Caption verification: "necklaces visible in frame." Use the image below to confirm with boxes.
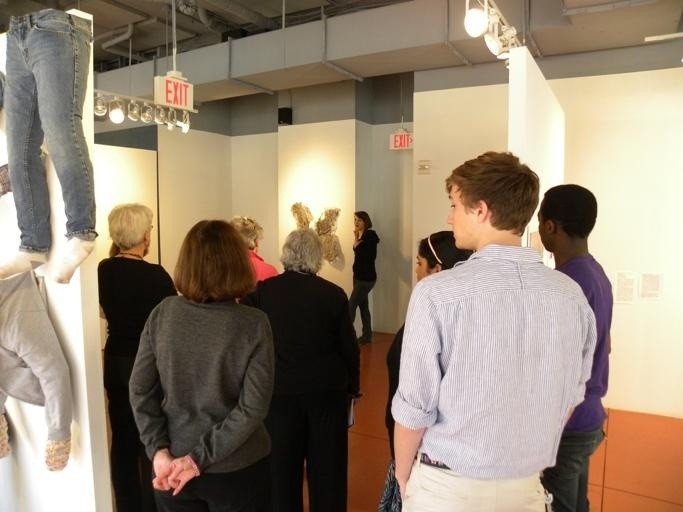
[119,252,143,259]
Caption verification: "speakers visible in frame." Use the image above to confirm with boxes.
[277,107,292,126]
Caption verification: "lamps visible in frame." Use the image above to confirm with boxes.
[464,0,518,70]
[93,90,191,134]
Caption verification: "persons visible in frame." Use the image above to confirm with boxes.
[129,219,275,510]
[97,204,178,512]
[378,230,474,511]
[227,216,279,306]
[243,228,360,510]
[391,151,598,512]
[106,240,149,510]
[347,211,379,344]
[538,184,614,512]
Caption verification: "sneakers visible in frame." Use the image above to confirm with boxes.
[357,337,371,344]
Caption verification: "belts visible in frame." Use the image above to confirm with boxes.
[413,452,449,470]
